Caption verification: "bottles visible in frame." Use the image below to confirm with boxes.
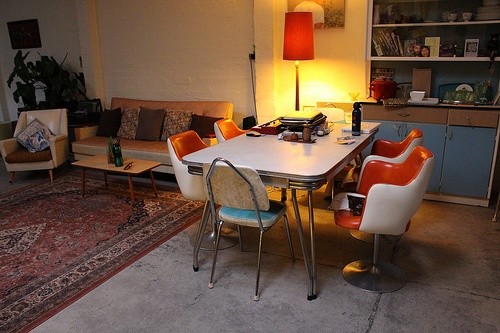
[351,102,362,136]
[302,124,310,142]
[112,141,123,167]
[291,132,298,140]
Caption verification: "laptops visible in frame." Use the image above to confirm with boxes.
[342,122,381,134]
[280,112,323,124]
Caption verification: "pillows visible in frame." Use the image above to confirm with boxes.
[97,106,224,141]
[16,118,56,153]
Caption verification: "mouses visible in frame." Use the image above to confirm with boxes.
[246,131,262,137]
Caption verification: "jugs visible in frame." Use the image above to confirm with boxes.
[475,80,490,102]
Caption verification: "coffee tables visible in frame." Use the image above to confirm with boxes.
[72,155,162,205]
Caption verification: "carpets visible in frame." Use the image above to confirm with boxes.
[0,170,222,333]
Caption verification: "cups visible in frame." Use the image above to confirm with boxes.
[409,90,426,102]
[462,13,472,22]
[446,13,457,22]
[443,90,475,101]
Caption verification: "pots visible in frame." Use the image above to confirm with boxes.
[369,77,399,100]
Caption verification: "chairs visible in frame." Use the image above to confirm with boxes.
[167,118,436,301]
[0,109,69,183]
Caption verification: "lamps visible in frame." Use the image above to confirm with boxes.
[283,12,314,111]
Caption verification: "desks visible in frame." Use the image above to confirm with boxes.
[182,123,380,301]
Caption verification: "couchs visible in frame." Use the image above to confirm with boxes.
[71,97,234,175]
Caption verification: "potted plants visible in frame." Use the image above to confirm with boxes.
[7,50,86,108]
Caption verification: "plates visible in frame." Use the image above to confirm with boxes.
[455,83,474,90]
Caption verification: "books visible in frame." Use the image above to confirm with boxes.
[373,28,404,57]
[278,110,328,128]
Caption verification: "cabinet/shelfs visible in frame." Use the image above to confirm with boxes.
[357,0,500,207]
